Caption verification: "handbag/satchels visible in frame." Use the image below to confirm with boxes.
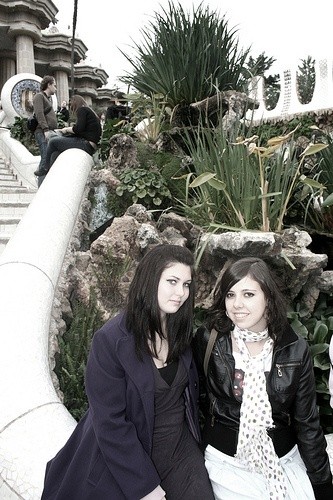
[27,113,39,132]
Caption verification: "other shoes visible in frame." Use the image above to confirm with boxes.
[34,168,47,176]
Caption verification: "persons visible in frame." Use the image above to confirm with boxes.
[57,107,69,122]
[41,244,213,500]
[33,75,58,188]
[34,95,102,176]
[104,92,131,120]
[193,257,333,500]
